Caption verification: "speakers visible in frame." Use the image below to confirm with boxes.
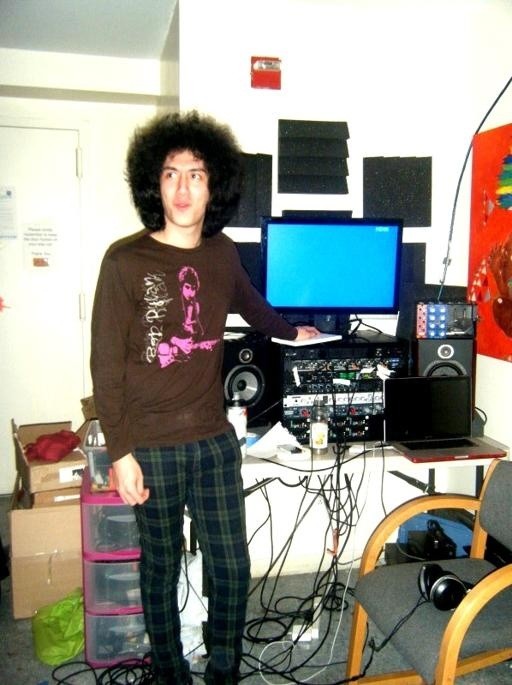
[222,338,280,428]
[413,339,477,423]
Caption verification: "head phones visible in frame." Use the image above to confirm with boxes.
[417,563,476,612]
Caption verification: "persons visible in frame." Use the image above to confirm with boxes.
[161,263,213,359]
[88,108,321,685]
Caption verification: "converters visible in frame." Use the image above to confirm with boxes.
[291,624,311,641]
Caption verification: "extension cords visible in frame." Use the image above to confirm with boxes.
[311,596,323,639]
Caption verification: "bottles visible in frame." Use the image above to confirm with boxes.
[224,392,248,460]
[308,399,329,456]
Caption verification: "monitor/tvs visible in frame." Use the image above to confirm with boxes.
[261,216,405,334]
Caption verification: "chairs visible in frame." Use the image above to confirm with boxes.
[350,456,510,683]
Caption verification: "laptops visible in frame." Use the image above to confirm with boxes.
[384,375,507,463]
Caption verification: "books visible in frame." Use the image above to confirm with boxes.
[272,332,343,349]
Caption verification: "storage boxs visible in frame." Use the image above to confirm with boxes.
[4,394,153,670]
